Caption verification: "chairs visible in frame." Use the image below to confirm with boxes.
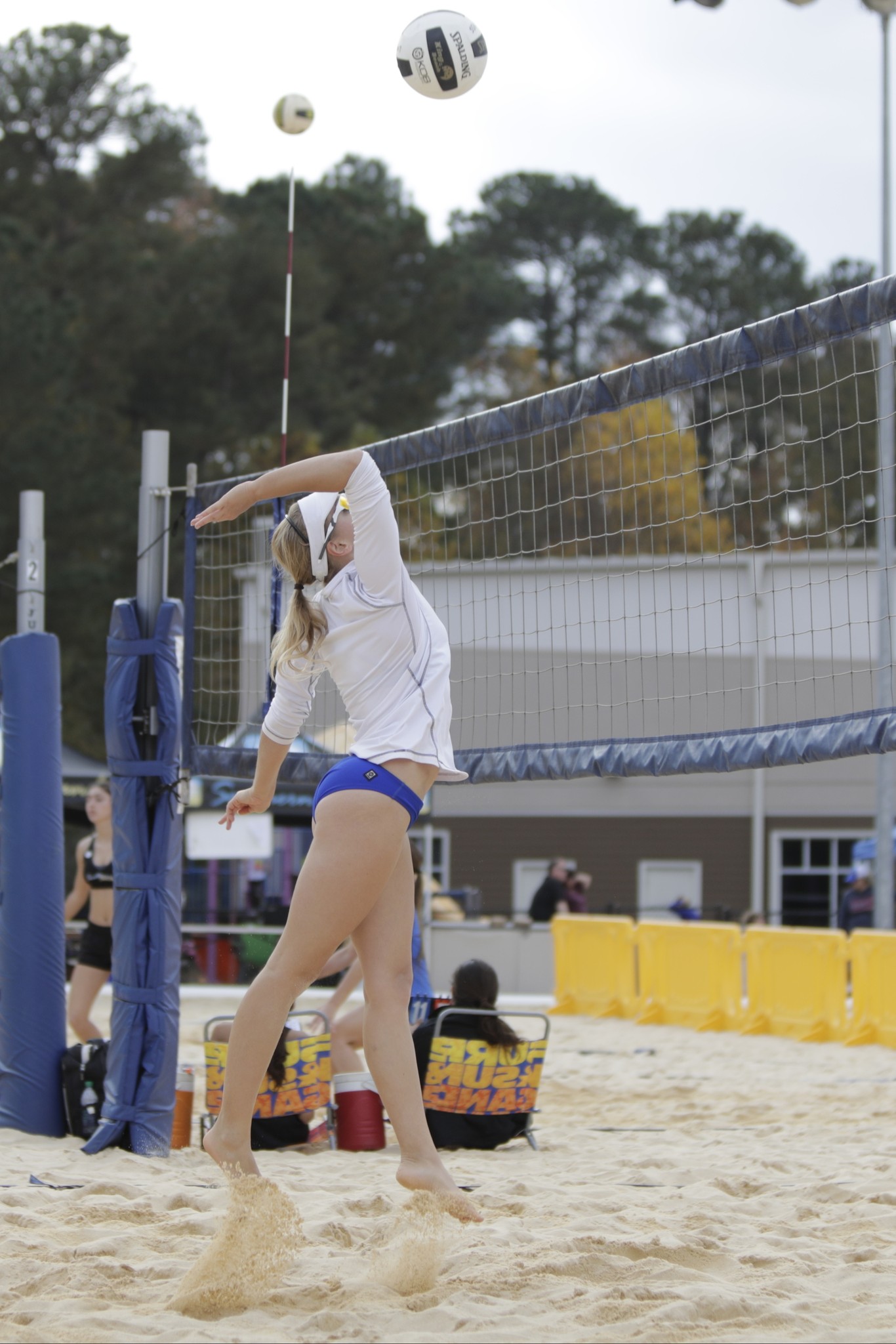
[423,1007,552,1153]
[199,1009,340,1154]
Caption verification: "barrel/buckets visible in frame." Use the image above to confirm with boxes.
[170,1067,194,1149]
[333,1072,386,1152]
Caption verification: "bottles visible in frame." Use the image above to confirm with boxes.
[81,1082,98,1132]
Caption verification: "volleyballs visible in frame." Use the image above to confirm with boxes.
[273,94,315,135]
[397,9,488,100]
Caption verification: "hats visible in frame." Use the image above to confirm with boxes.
[297,492,339,583]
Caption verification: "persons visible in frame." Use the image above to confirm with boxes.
[232,892,289,983]
[305,844,432,1075]
[836,861,873,931]
[411,958,529,1152]
[61,777,113,1044]
[527,855,593,923]
[667,895,699,919]
[189,450,485,1225]
[745,914,765,924]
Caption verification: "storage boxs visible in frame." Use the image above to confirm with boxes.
[333,1071,387,1152]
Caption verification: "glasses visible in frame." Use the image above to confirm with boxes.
[318,493,349,564]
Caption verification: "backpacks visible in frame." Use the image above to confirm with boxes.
[59,1038,111,1139]
